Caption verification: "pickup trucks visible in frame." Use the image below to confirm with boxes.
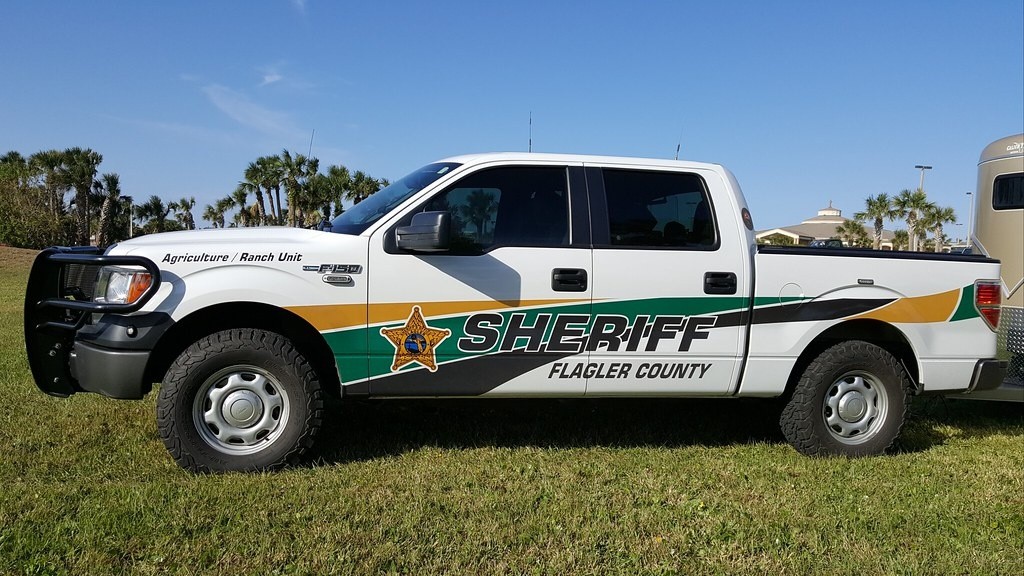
[22,152,1002,477]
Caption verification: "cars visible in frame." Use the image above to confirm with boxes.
[809,239,843,248]
[945,133,1024,402]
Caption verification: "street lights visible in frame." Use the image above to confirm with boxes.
[915,165,932,218]
[956,224,963,243]
[966,192,974,248]
